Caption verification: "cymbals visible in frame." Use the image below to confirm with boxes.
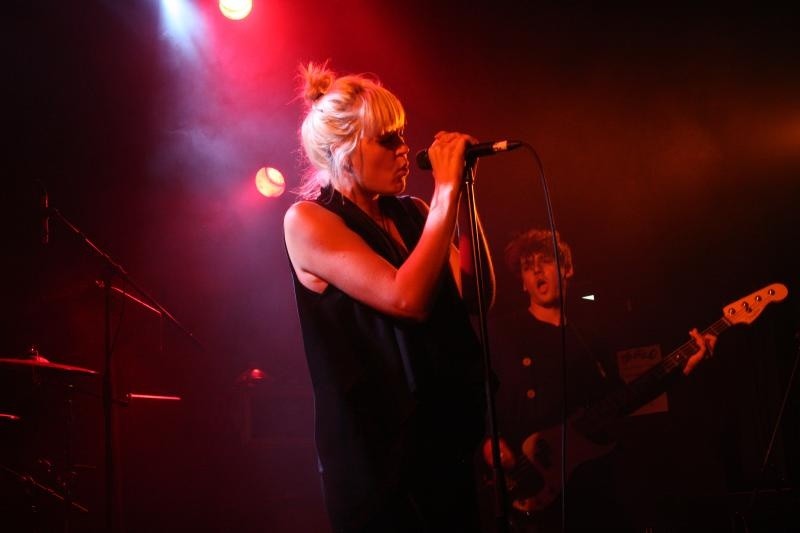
[0,355,100,375]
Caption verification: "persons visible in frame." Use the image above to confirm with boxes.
[282,60,497,532]
[486,229,707,533]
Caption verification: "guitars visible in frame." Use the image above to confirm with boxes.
[503,278,788,513]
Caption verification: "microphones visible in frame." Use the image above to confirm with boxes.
[417,140,522,170]
[40,187,49,243]
